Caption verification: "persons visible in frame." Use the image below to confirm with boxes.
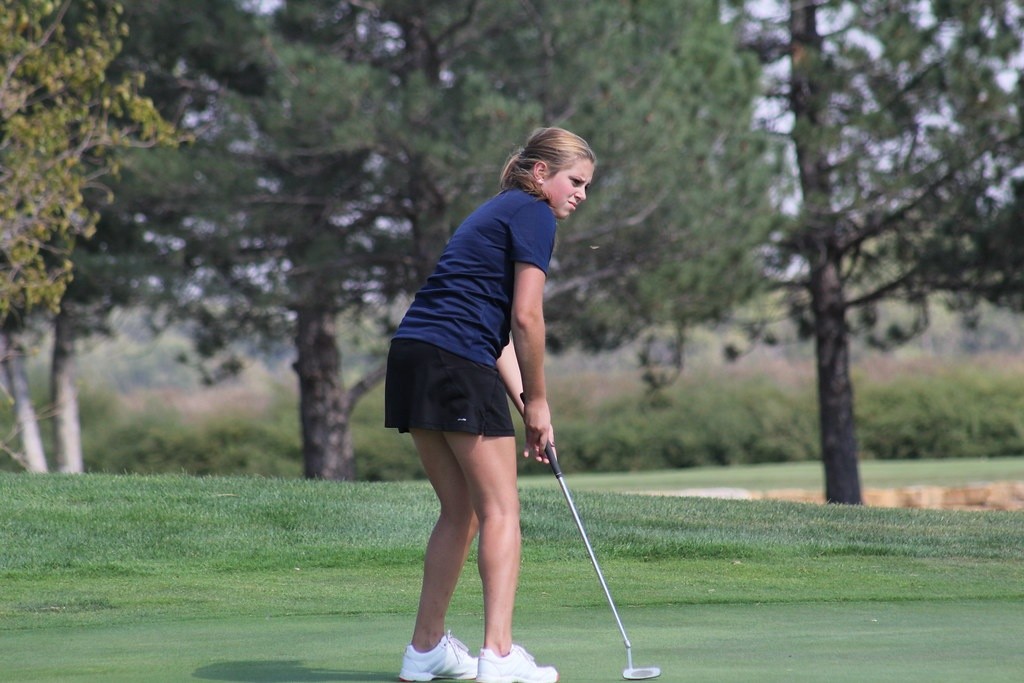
[384,127,596,683]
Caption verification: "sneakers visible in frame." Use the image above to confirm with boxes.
[397,628,480,682]
[476,644,559,683]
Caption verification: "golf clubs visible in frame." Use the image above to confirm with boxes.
[519,391,663,679]
